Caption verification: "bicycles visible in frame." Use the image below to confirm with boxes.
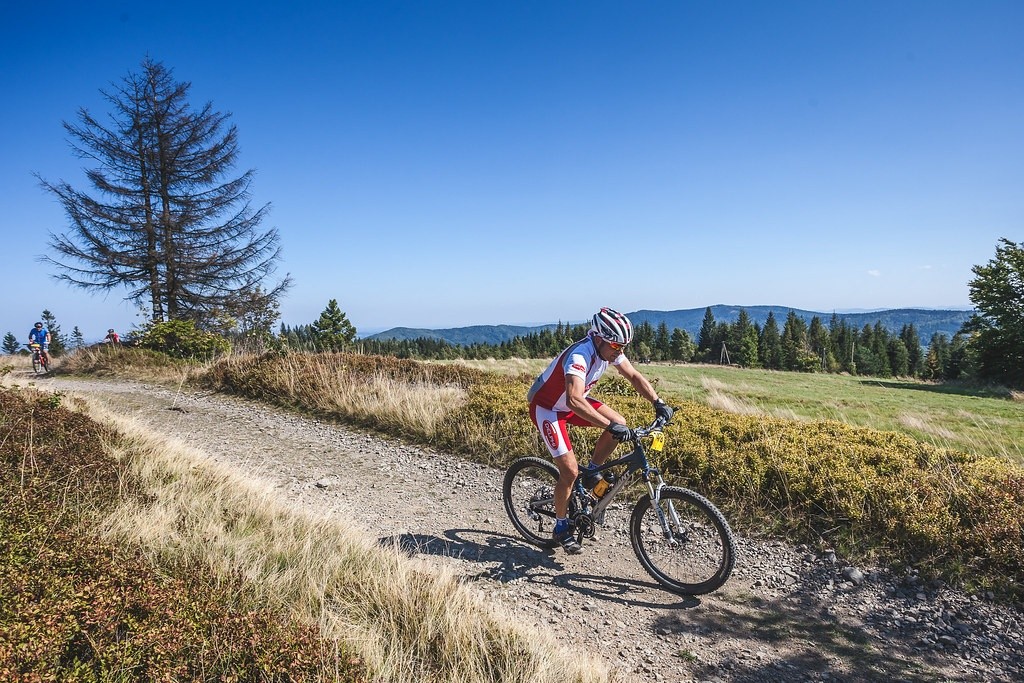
[503,407,736,597]
[29,341,50,374]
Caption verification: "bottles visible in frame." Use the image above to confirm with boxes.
[590,470,616,499]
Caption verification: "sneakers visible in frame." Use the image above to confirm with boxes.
[582,468,610,499]
[553,527,583,554]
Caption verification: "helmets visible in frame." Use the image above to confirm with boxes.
[588,307,634,345]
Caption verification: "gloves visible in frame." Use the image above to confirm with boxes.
[606,422,631,444]
[654,398,673,421]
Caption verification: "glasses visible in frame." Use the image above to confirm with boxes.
[610,343,623,351]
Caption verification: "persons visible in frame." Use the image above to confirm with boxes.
[102,328,119,343]
[524,305,675,556]
[28,322,51,365]
